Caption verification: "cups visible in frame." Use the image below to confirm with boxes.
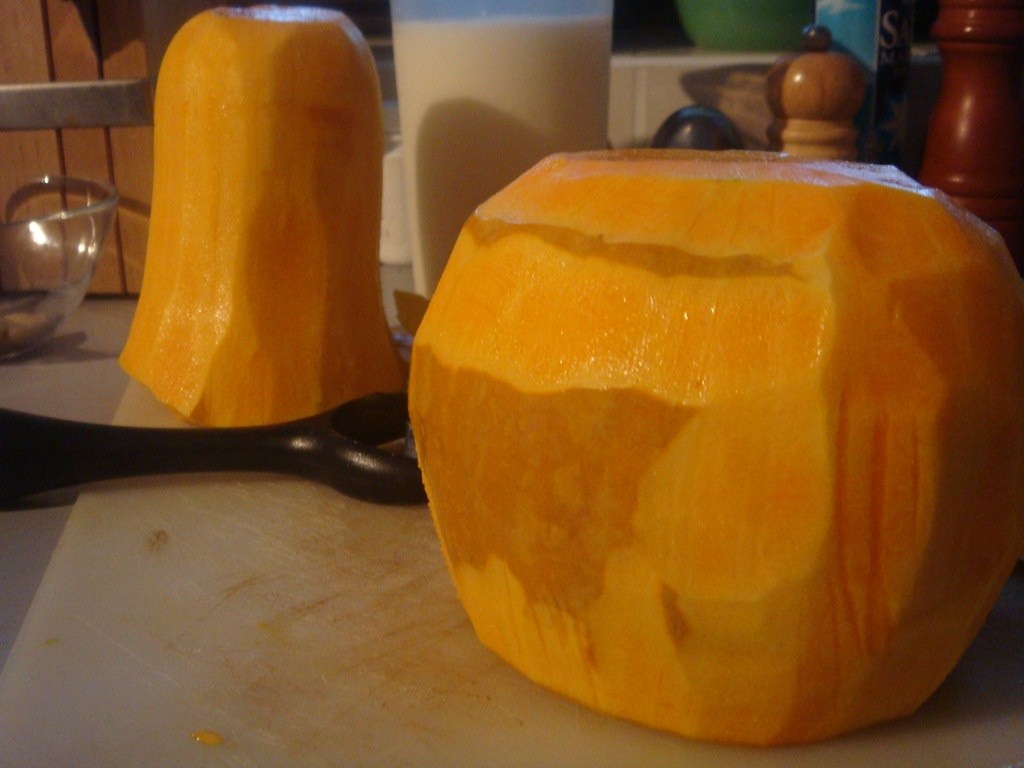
[387,0,612,297]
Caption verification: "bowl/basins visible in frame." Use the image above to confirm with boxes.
[675,0,814,50]
[0,167,121,362]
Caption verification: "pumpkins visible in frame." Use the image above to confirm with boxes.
[121,5,405,427]
[400,148,1024,745]
